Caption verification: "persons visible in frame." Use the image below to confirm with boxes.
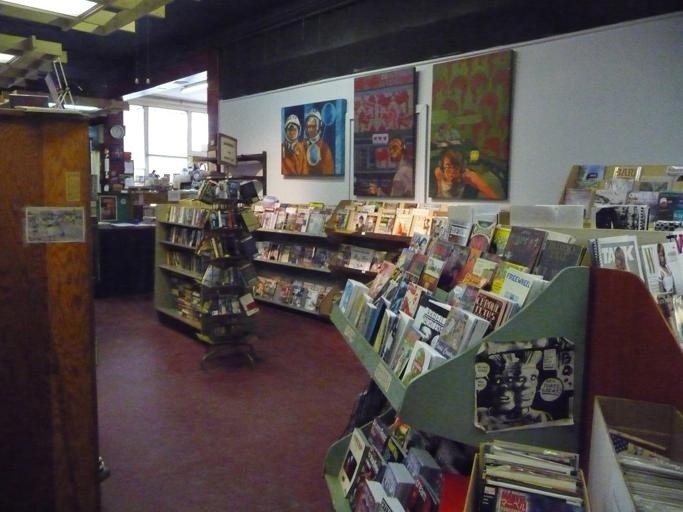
[432,152,495,201]
[353,214,365,236]
[654,244,681,328]
[512,352,554,423]
[300,107,337,174]
[611,244,629,270]
[366,136,412,195]
[280,114,308,177]
[297,212,307,232]
[476,353,515,428]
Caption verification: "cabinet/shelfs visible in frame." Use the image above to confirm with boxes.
[0,103,111,510]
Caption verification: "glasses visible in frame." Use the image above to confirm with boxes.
[441,165,460,174]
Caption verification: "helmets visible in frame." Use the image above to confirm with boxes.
[305,111,323,143]
[285,115,302,143]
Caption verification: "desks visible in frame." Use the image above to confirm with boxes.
[95,219,155,295]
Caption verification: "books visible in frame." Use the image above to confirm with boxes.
[335,213,586,512]
[249,198,449,316]
[563,163,683,340]
[161,165,261,360]
[610,426,681,512]
